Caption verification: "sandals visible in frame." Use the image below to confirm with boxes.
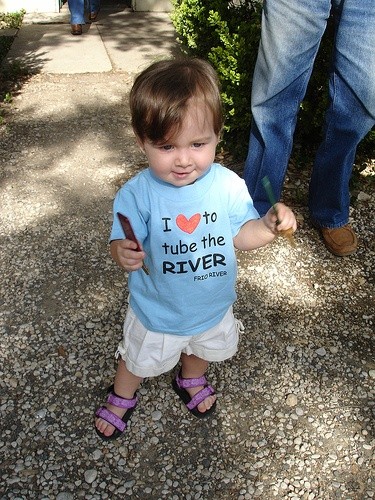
[172,368,218,418]
[95,385,138,439]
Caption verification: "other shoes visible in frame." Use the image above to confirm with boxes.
[320,226,357,256]
[88,8,100,19]
[71,24,82,35]
